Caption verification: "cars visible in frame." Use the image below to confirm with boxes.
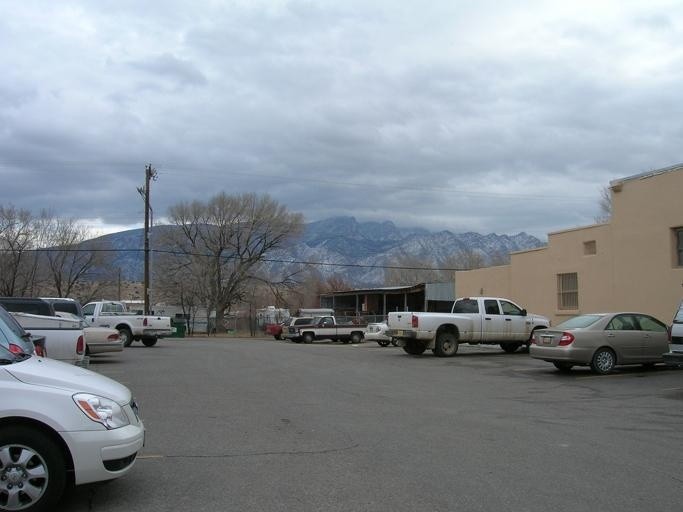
[0,296,124,371]
[0,344,147,511]
[529,312,670,376]
[663,298,682,368]
[364,317,406,347]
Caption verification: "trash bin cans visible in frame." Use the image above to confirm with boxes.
[171,318,186,338]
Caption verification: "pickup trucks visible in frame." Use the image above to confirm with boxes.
[262,308,367,344]
[80,299,177,347]
[385,297,551,357]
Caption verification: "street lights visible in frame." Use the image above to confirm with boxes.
[134,186,153,315]
